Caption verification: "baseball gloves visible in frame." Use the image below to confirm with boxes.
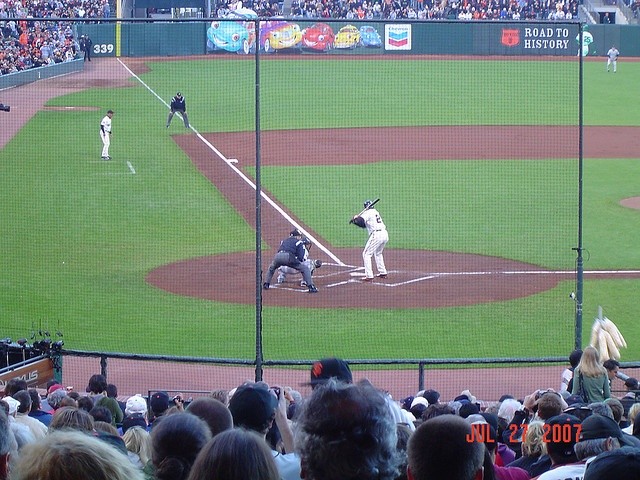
[316,260,321,268]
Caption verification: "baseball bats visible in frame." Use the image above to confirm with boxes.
[349,198,380,224]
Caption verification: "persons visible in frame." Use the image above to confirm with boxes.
[576,24,598,56]
[1,346,639,479]
[210,1,579,20]
[99,110,113,160]
[624,0,640,18]
[167,92,189,128]
[603,13,611,24]
[263,229,319,293]
[351,200,389,281]
[278,240,315,287]
[1,0,110,76]
[607,45,620,72]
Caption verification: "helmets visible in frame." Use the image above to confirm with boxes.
[364,201,373,208]
[303,237,311,254]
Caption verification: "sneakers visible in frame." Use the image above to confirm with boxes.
[544,413,582,454]
[278,277,287,283]
[362,277,375,282]
[101,156,112,160]
[308,285,318,293]
[263,282,270,290]
[301,281,307,287]
[375,273,388,279]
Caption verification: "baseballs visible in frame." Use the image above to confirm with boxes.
[593,50,597,54]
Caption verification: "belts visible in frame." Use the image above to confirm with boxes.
[376,229,381,231]
[280,251,291,253]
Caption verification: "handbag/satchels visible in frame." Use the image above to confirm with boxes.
[578,389,590,403]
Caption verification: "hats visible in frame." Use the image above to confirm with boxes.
[122,413,146,434]
[47,383,63,397]
[107,110,114,114]
[569,349,583,361]
[400,397,415,407]
[298,358,351,385]
[229,381,278,422]
[3,396,20,415]
[290,229,301,236]
[563,403,592,420]
[424,389,441,404]
[150,391,169,411]
[177,92,181,96]
[585,445,640,480]
[411,397,429,408]
[566,395,584,404]
[625,378,637,387]
[125,396,147,411]
[576,414,635,446]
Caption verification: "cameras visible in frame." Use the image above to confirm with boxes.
[271,388,281,398]
[537,388,552,397]
[171,396,182,405]
